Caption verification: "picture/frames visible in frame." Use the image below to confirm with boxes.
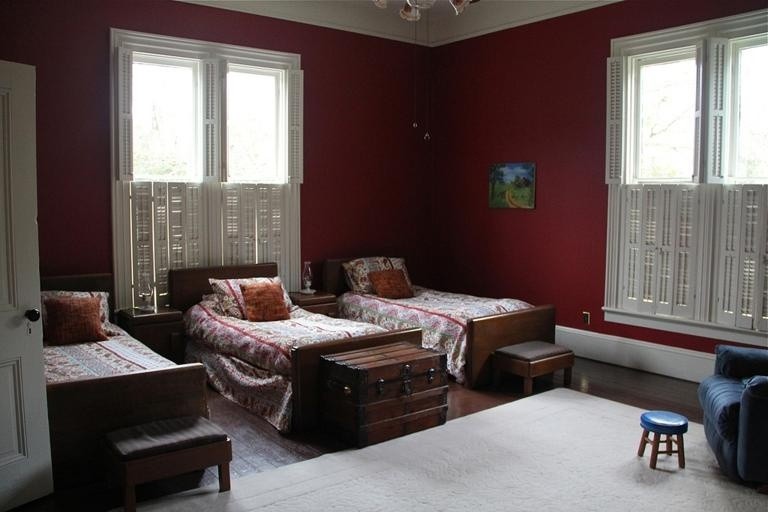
[488,162,538,210]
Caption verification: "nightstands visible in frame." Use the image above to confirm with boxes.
[116,307,187,364]
[290,288,338,317]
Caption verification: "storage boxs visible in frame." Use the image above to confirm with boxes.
[319,341,449,448]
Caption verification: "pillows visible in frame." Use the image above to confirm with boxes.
[48,296,110,344]
[240,283,290,321]
[208,276,292,319]
[343,257,413,293]
[369,269,414,299]
[42,290,120,341]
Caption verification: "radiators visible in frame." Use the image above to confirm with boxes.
[120,181,302,306]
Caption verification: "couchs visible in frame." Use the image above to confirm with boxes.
[697,345,768,493]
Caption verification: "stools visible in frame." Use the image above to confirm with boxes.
[105,414,232,510]
[496,341,575,395]
[637,410,689,470]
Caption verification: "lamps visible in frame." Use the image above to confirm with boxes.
[375,0,471,21]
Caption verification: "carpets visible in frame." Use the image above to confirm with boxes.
[130,387,768,512]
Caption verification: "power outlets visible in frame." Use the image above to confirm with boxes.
[582,312,590,325]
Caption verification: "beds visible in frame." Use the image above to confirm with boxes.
[167,263,423,438]
[40,272,209,489]
[325,253,556,390]
